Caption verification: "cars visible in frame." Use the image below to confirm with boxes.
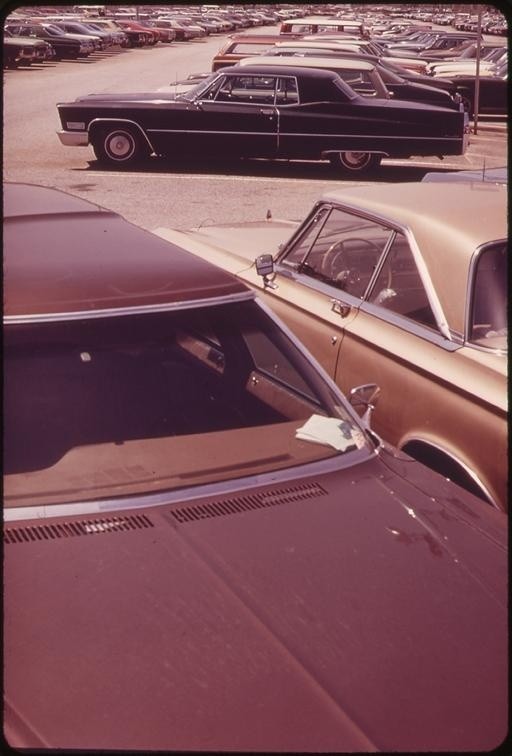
[56,65,473,175]
[1,182,512,751]
[151,186,511,516]
